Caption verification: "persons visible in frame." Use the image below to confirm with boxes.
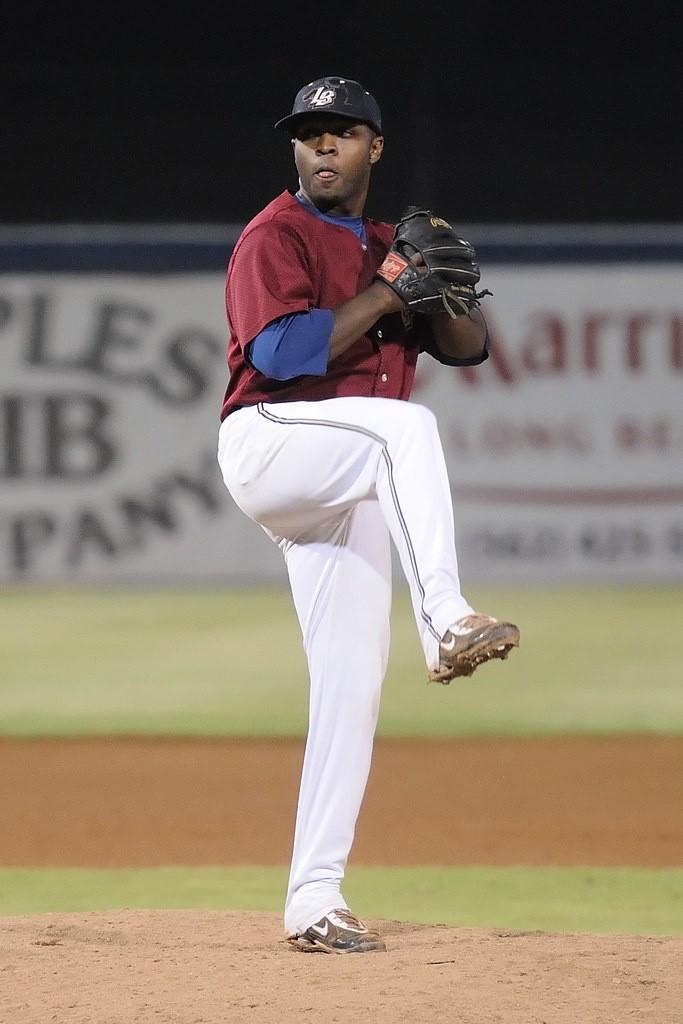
[216,76,520,955]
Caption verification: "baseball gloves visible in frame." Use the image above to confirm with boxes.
[370,203,495,339]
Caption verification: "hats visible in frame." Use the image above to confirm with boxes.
[271,76,383,141]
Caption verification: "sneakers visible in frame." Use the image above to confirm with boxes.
[429,614,519,684]
[288,909,386,956]
